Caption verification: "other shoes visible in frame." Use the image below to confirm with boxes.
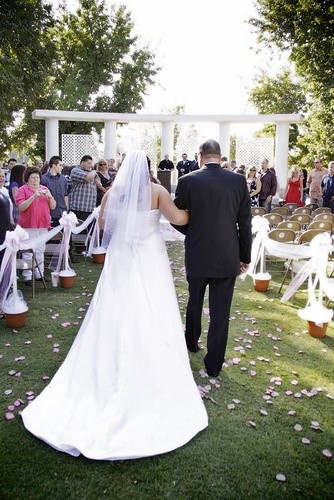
[71,259,80,263]
[35,277,49,282]
[25,280,32,286]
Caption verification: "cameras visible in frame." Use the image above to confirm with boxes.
[41,190,47,195]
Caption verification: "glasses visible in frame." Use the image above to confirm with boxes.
[100,164,106,166]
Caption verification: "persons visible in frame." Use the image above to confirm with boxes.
[0,152,126,288]
[97,152,189,364]
[168,139,252,377]
[157,154,334,214]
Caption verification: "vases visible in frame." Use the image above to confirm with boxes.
[59,276,76,289]
[5,312,26,328]
[307,320,328,338]
[91,253,106,264]
[254,279,270,292]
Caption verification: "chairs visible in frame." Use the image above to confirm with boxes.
[250,203,334,306]
[44,226,65,271]
[15,248,47,300]
[70,219,89,262]
